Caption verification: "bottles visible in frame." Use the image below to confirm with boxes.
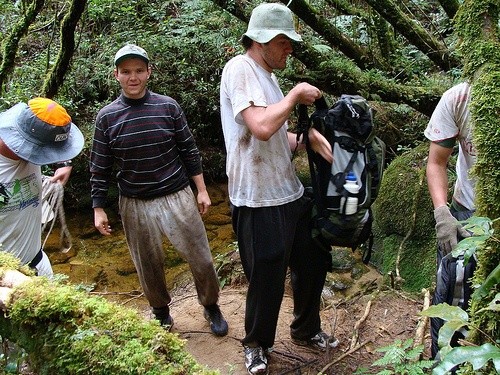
[340,172,359,215]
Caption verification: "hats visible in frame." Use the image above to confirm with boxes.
[238,2,303,48]
[114,44,150,64]
[0,97,84,165]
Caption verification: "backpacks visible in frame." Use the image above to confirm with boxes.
[292,92,385,253]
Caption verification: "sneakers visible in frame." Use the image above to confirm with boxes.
[291,331,339,351]
[244,346,269,375]
[203,308,229,337]
[157,316,174,331]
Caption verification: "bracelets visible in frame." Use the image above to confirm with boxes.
[57,162,73,167]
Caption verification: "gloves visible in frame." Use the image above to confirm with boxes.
[434,205,471,255]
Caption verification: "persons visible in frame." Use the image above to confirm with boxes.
[219,3,340,375]
[89,43,229,336]
[423,80,478,360]
[0,97,85,277]
